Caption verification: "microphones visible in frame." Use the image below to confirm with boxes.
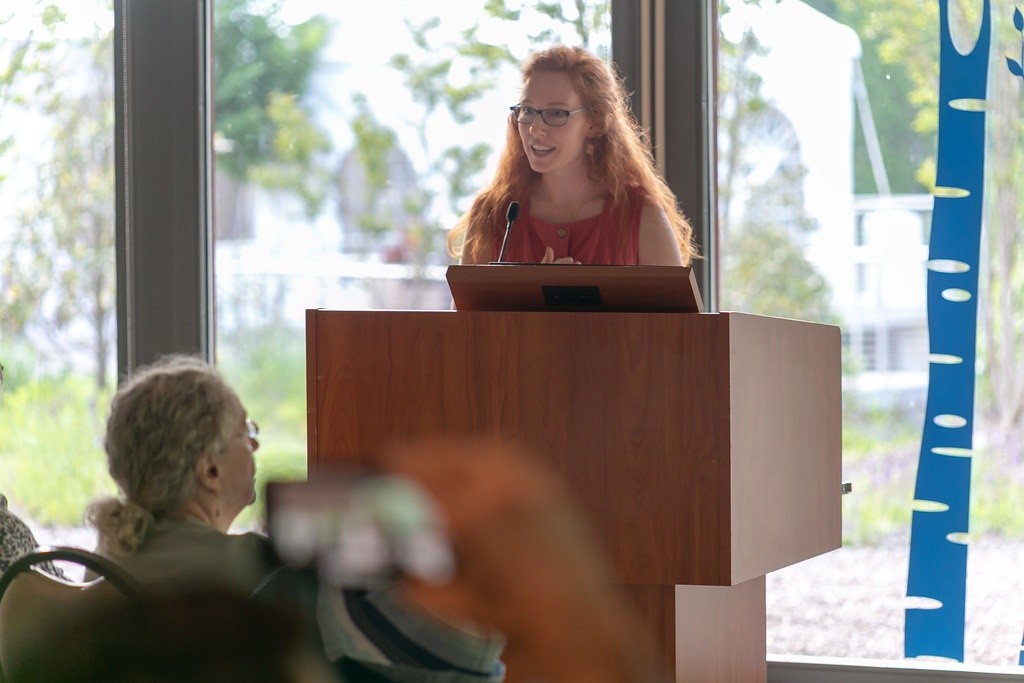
[497,202,520,263]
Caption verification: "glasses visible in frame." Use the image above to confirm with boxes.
[510,102,586,127]
[216,419,259,454]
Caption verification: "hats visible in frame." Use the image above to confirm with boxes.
[316,579,508,683]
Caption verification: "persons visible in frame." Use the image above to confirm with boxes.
[0,363,74,583]
[83,360,316,627]
[450,45,691,266]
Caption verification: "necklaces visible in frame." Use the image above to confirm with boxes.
[553,203,587,219]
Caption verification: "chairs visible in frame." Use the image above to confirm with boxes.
[0,531,505,683]
